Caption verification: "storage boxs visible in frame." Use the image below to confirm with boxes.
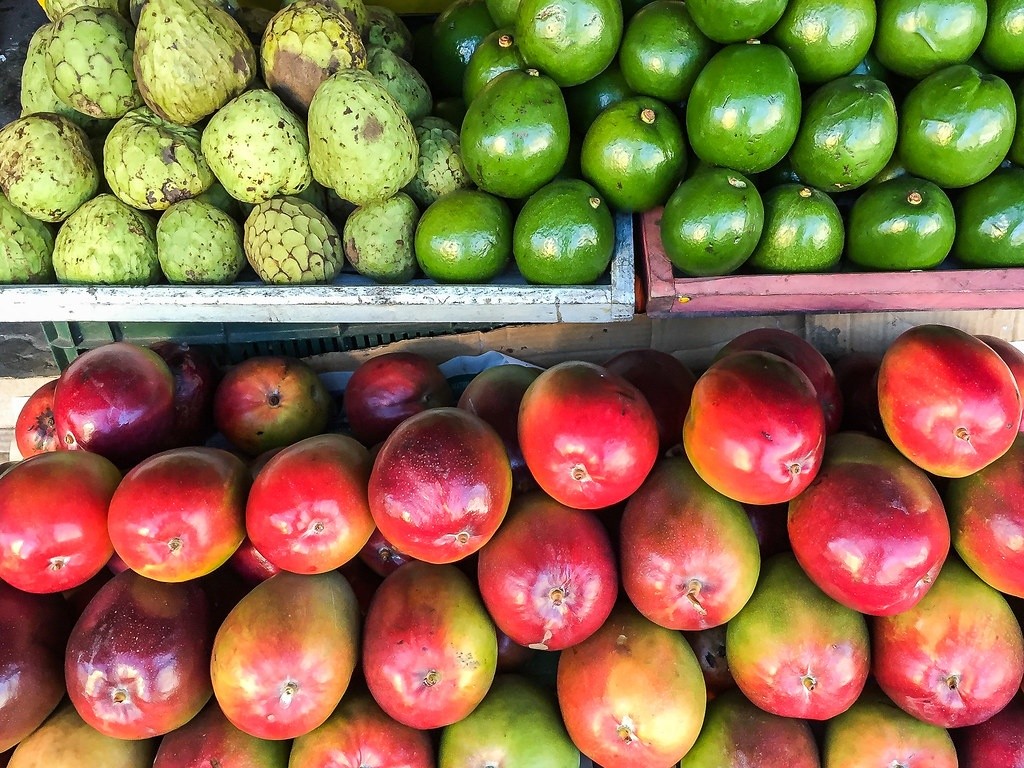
[0,0,1024,430]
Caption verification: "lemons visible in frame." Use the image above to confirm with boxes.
[411,0,1024,289]
